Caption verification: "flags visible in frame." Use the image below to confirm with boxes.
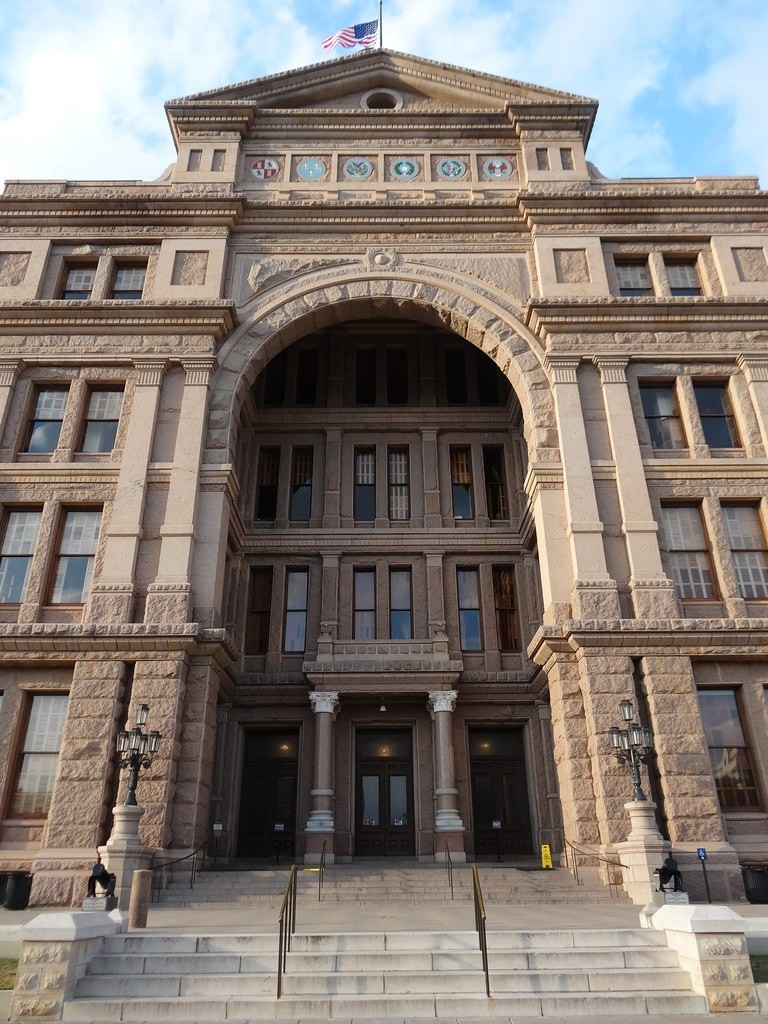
[321,19,379,54]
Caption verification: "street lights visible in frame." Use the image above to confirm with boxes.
[608,699,653,801]
[117,703,162,806]
